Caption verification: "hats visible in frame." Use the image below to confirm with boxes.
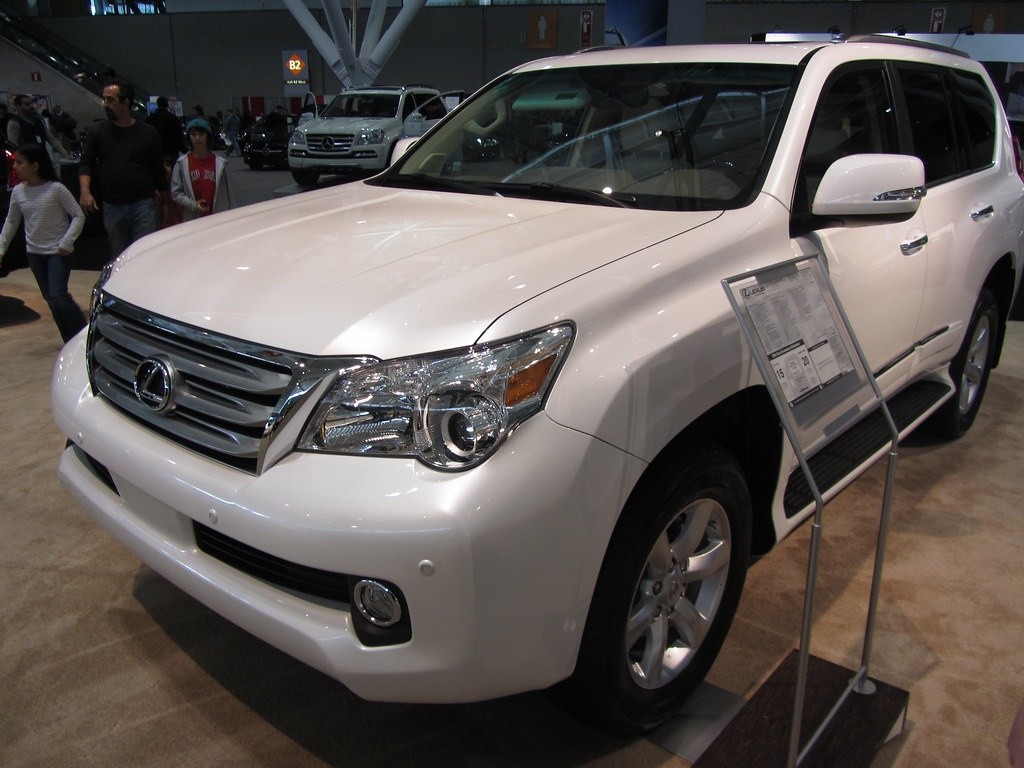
[185,119,212,134]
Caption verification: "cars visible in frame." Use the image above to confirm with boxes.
[49,11,1024,752]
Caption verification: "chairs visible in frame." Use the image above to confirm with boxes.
[740,95,854,212]
[596,98,692,190]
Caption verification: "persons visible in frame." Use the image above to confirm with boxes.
[78,80,166,260]
[154,160,183,230]
[0,52,290,163]
[0,143,88,345]
[170,118,233,222]
